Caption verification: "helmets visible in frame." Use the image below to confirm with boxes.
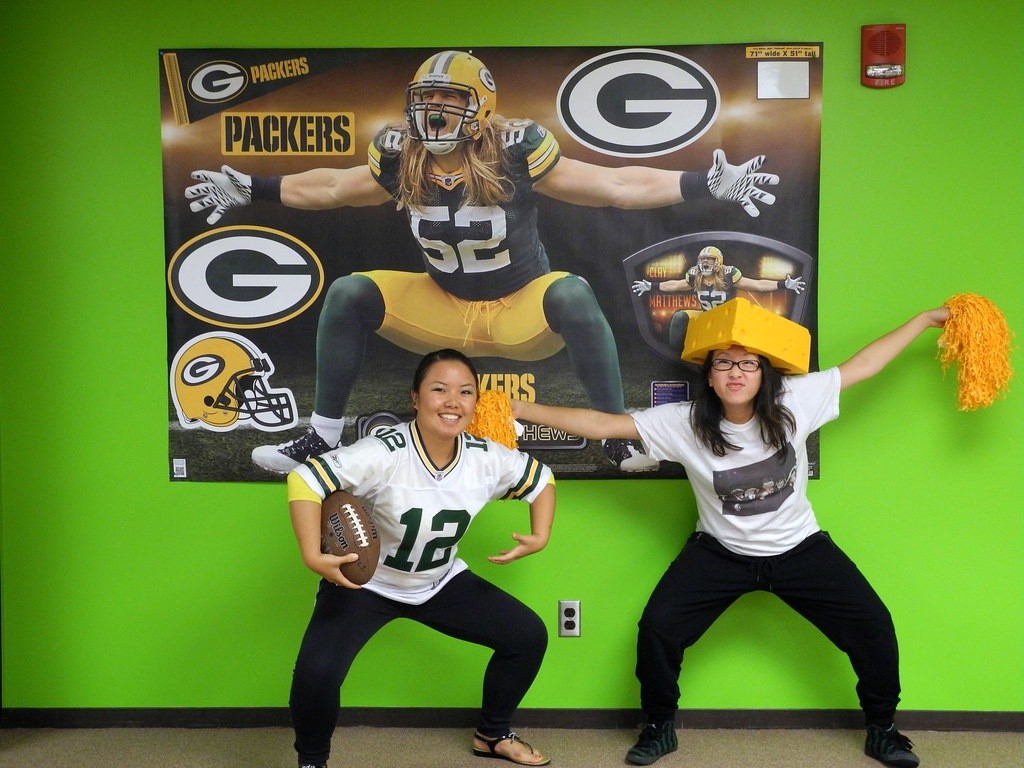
[410,50,497,143]
[698,246,723,273]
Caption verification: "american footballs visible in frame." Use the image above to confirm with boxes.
[317,490,382,589]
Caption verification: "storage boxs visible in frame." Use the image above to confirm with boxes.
[681,297,811,375]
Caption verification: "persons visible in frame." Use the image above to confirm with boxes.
[184,49,809,480]
[508,297,952,768]
[287,347,556,768]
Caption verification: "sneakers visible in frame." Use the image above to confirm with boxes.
[626,720,678,765]
[602,438,660,472]
[251,426,343,475]
[865,723,920,768]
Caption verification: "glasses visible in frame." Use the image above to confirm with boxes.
[710,359,761,372]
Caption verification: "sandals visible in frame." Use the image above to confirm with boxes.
[471,730,551,766]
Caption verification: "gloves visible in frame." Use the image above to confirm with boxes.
[785,275,806,295]
[632,279,652,297]
[706,149,780,218]
[185,165,252,225]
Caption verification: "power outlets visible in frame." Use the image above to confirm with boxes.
[558,600,581,637]
